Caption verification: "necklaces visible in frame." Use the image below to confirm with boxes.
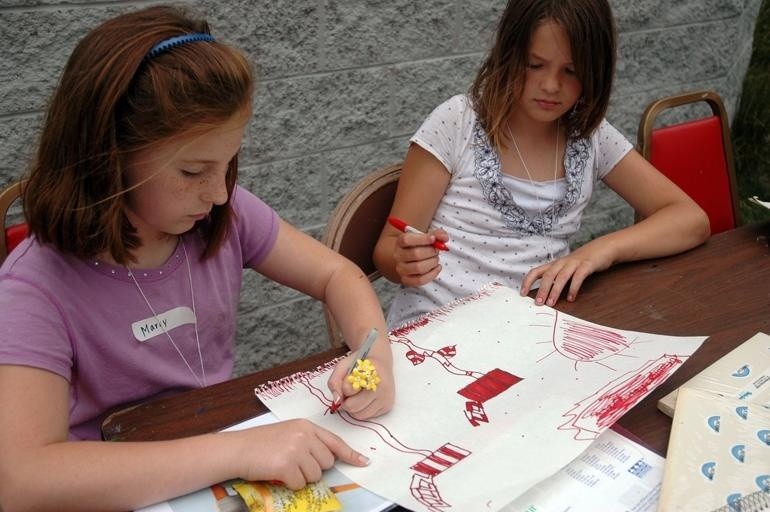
[502,117,561,264]
[119,236,209,389]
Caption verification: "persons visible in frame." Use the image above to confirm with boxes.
[0,1,398,510]
[372,0,711,332]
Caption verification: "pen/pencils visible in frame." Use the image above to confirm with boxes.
[331,328,380,414]
[386,213,451,254]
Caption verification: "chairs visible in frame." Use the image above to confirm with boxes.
[1,179,43,259]
[634,94,739,240]
[321,164,402,349]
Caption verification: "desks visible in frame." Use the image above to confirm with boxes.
[102,224,770,512]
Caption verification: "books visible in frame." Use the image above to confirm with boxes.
[656,331,770,421]
[129,411,398,512]
[499,427,667,512]
[653,386,769,511]
